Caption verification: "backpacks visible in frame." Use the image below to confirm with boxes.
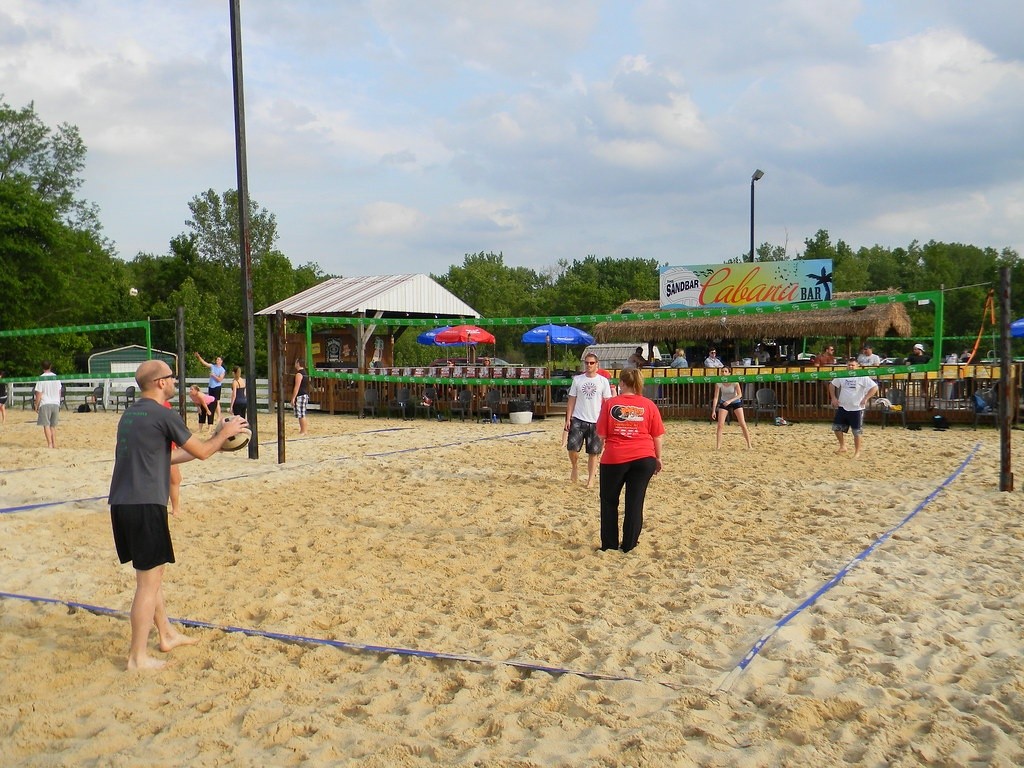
[934,415,950,429]
[77,403,92,412]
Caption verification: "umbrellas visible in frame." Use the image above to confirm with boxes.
[416,324,496,367]
[520,322,596,372]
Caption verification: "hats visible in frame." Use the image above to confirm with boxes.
[914,343,926,352]
[864,344,873,350]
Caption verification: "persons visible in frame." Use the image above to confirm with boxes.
[163,352,247,519]
[712,366,753,450]
[809,343,974,368]
[563,354,612,490]
[829,358,879,459]
[0,373,8,425]
[478,356,491,397]
[34,360,62,450]
[580,342,772,397]
[446,359,458,398]
[594,367,666,553]
[107,360,249,673]
[291,358,308,436]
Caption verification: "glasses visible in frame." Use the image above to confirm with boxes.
[710,352,716,354]
[154,373,175,382]
[828,348,835,351]
[585,361,597,365]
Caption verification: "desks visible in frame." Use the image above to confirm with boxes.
[825,363,861,366]
[644,363,672,369]
[732,364,765,367]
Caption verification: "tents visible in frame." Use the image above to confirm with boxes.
[591,287,914,342]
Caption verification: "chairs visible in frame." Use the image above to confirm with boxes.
[387,388,502,425]
[700,361,706,368]
[85,387,106,413]
[58,386,68,411]
[363,388,380,419]
[116,386,135,412]
[752,388,776,427]
[23,387,36,410]
[880,389,907,429]
[973,388,998,431]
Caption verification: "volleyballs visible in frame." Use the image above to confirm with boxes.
[129,288,138,297]
[215,417,251,452]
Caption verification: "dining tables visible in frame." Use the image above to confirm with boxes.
[709,399,732,425]
